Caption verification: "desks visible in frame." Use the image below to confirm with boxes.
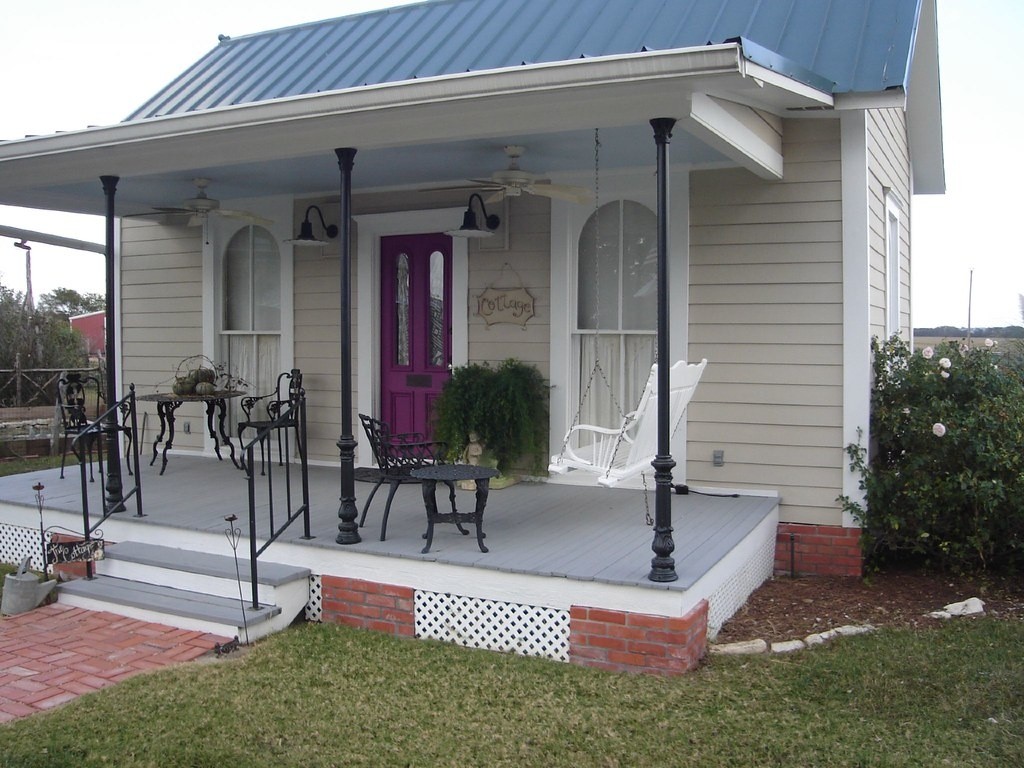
[409,464,500,554]
[134,390,244,476]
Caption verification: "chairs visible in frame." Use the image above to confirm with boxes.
[56,374,133,482]
[238,369,303,476]
[359,414,470,541]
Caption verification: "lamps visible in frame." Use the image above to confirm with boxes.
[442,193,500,239]
[283,205,338,248]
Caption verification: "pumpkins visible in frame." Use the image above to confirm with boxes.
[169,364,216,395]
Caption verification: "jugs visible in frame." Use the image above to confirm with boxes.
[0,557,58,616]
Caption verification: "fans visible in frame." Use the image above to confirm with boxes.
[149,177,278,228]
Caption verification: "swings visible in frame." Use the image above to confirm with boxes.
[548,127,707,489]
